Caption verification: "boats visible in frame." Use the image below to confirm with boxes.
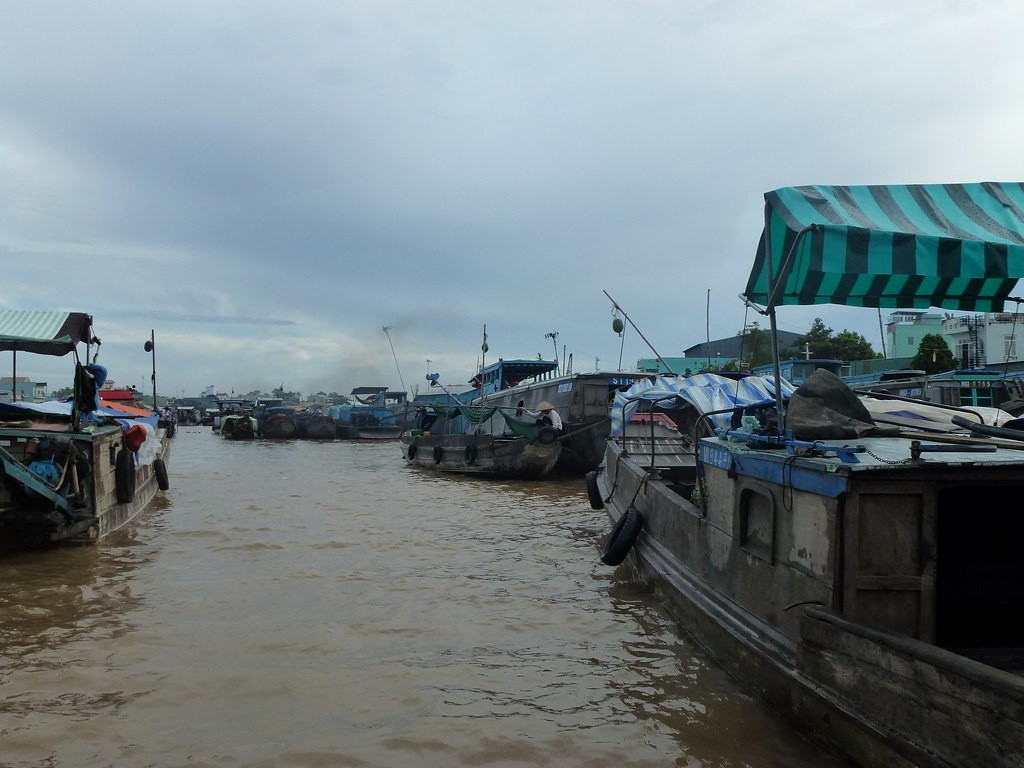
[402,181,1024,768]
[167,386,412,442]
[1,308,177,546]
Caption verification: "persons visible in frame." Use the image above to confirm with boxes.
[516,402,563,436]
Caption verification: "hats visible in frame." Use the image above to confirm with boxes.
[536,401,555,411]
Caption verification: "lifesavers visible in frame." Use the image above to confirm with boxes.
[585,470,604,511]
[463,444,477,465]
[114,448,137,507]
[154,458,169,491]
[432,444,442,465]
[407,444,416,460]
[537,425,557,445]
[600,505,645,567]
